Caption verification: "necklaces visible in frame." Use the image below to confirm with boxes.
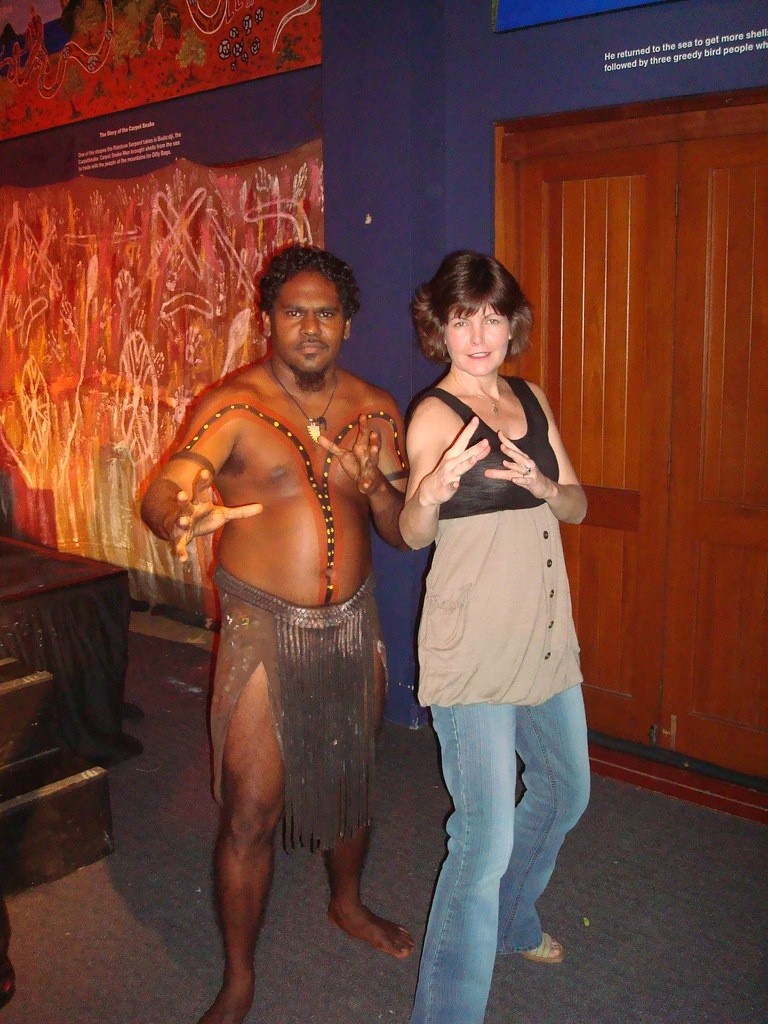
[450,371,505,415]
[272,357,340,447]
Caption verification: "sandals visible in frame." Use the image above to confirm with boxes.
[518,932,566,964]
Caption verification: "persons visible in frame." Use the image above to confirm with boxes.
[136,244,417,1022]
[395,248,598,1024]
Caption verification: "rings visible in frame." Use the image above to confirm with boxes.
[523,465,530,475]
